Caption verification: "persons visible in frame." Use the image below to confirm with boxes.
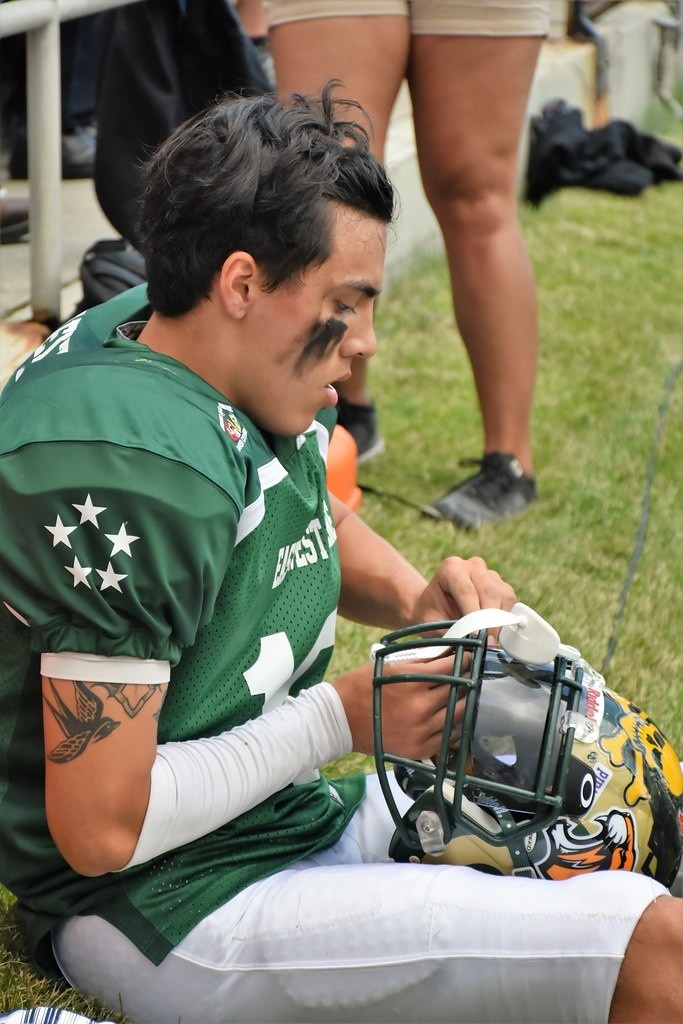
[0,0,553,532]
[0,82,683,1023]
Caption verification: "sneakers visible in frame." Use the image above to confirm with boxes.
[335,396,385,464]
[433,451,537,526]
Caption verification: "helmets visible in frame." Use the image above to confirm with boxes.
[389,643,683,897]
